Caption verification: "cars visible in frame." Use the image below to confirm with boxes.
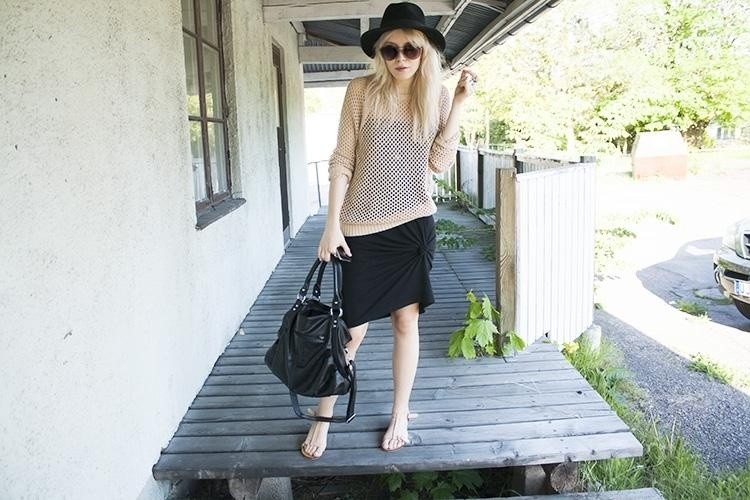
[714,221,750,321]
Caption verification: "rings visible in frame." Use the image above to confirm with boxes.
[471,75,479,83]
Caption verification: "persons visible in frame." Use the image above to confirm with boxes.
[299,2,479,461]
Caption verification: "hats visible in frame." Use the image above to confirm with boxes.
[360,2,447,59]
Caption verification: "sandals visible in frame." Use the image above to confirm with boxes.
[381,411,411,453]
[301,409,326,458]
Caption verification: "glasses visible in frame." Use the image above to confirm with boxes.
[380,43,423,60]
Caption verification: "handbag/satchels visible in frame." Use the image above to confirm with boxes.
[265,295,353,397]
